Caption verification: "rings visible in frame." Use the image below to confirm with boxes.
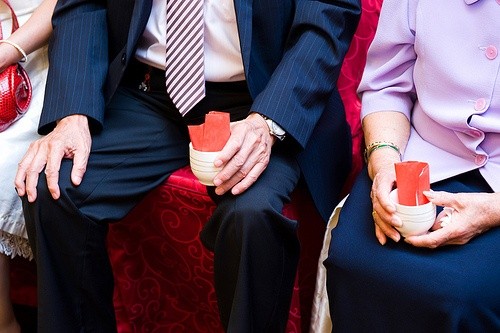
[372,211,376,215]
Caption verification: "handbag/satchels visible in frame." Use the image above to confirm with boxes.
[0,63,33,132]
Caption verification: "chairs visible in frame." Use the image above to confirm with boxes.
[107,0,383,333]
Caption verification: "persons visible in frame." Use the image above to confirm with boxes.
[0,0,58,333]
[16,0,363,333]
[321,0,500,333]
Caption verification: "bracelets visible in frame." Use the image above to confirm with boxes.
[0,40,27,63]
[364,140,402,161]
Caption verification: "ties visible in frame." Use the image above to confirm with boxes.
[167,0,206,117]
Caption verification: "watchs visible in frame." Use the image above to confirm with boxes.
[262,114,287,141]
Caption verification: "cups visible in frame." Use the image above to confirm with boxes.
[387,188,436,238]
[189,142,229,186]
